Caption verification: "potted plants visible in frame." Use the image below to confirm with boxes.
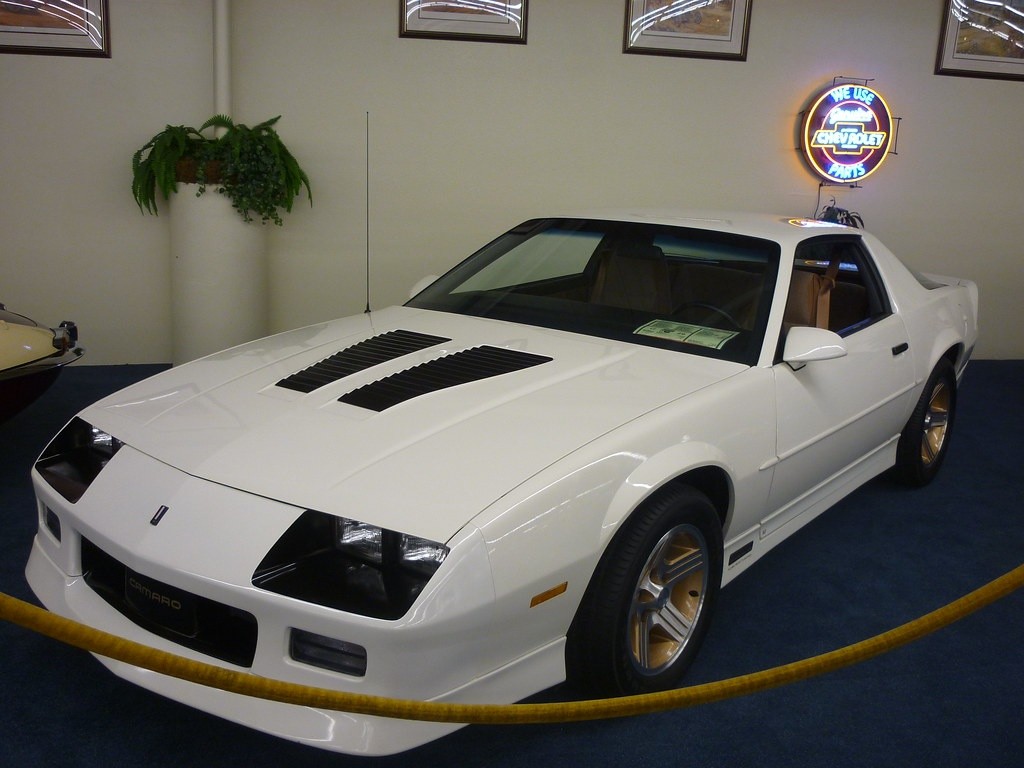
[131,113,313,226]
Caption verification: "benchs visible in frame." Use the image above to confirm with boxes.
[671,263,868,332]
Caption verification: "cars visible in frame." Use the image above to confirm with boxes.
[21,213,980,756]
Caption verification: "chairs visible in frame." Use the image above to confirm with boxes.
[590,245,673,315]
[744,268,821,336]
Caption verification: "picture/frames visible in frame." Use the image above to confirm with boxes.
[934,0,1024,82]
[0,0,112,59]
[398,0,528,45]
[622,0,753,62]
